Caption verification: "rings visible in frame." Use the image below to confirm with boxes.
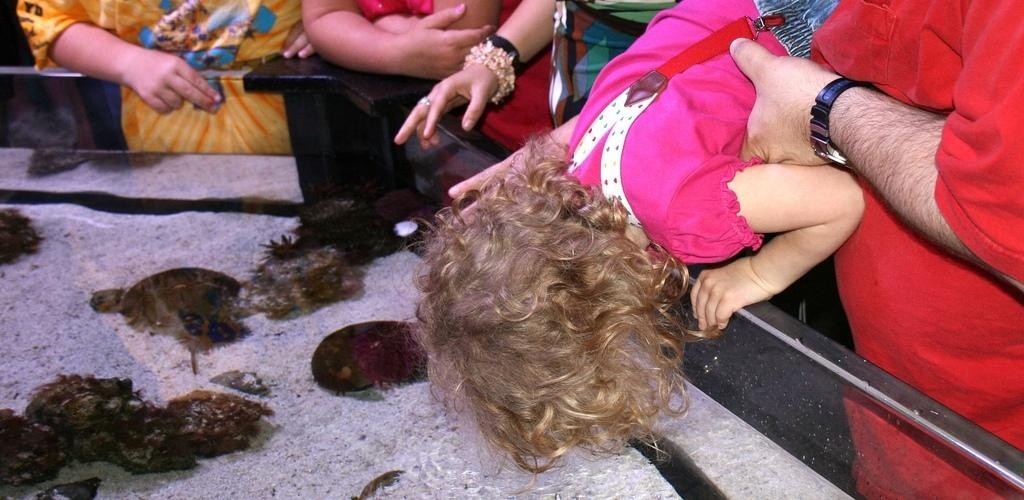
[419,95,433,109]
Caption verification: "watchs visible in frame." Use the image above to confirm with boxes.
[809,78,882,168]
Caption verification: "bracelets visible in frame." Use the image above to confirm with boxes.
[479,31,523,76]
[463,41,515,107]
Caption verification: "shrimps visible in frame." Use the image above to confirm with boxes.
[350,467,408,500]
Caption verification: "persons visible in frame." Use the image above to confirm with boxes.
[728,1,1024,499]
[394,0,688,152]
[283,0,554,154]
[409,1,865,477]
[16,0,315,156]
